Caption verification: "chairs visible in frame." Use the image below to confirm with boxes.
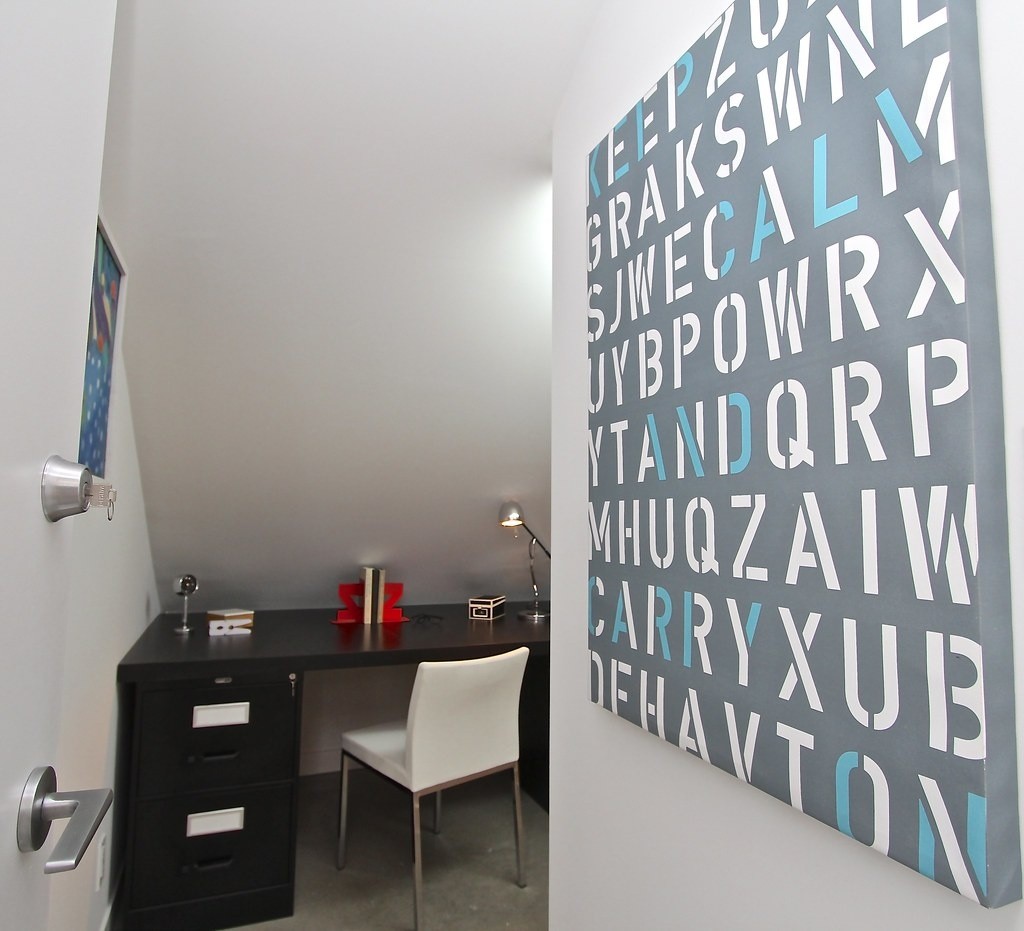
[332,646,532,931]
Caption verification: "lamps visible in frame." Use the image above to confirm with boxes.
[498,500,551,619]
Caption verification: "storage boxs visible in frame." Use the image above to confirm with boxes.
[207,608,255,628]
[468,595,506,621]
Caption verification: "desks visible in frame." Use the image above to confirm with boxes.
[110,600,550,931]
